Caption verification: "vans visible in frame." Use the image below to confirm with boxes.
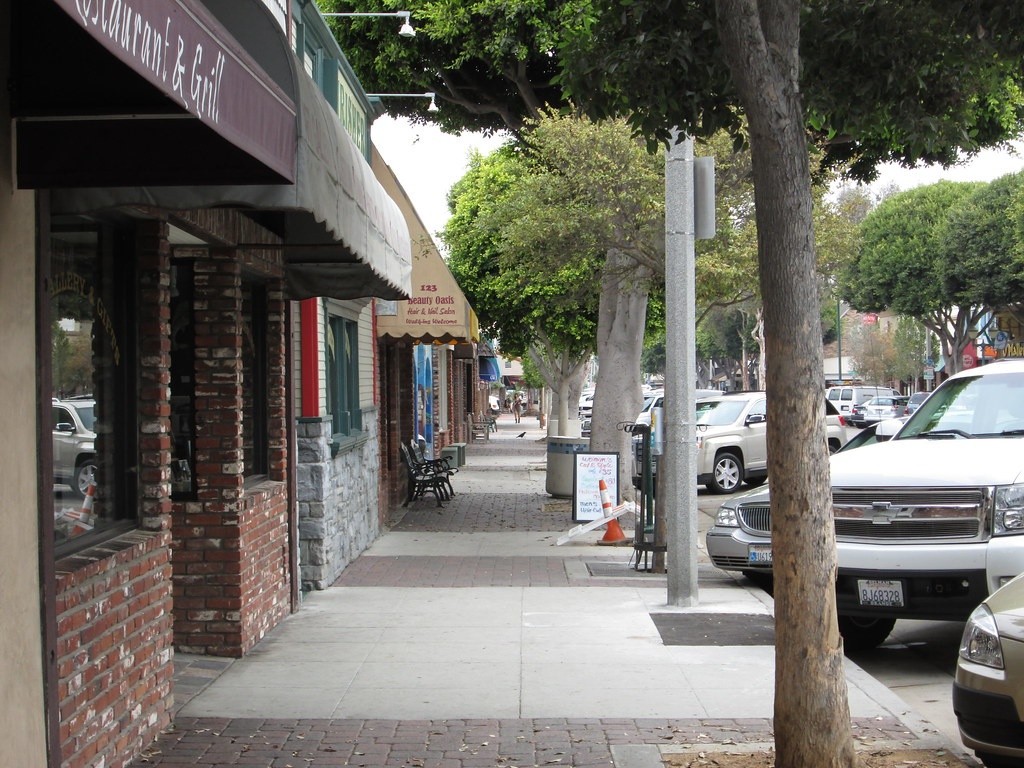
[631,387,723,437]
[828,385,902,427]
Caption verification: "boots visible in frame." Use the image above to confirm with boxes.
[515,419,517,424]
[518,418,520,422]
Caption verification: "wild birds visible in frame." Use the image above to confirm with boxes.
[516,432,526,438]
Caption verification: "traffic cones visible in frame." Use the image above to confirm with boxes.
[598,479,635,549]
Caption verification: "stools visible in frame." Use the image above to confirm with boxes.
[441,443,466,469]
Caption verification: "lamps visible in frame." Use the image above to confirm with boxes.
[365,92,439,112]
[321,11,415,37]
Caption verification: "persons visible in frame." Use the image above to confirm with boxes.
[513,393,527,423]
[505,397,512,412]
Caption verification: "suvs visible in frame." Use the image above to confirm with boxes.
[52,396,97,503]
[631,390,848,493]
[827,360,1024,650]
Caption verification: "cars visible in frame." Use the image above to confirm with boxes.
[706,415,971,583]
[578,391,594,435]
[489,395,501,413]
[850,391,950,430]
[955,572,1024,768]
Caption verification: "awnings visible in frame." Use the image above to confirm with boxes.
[52,50,413,301]
[370,140,479,346]
[453,343,475,359]
[14,0,303,190]
[477,343,497,358]
[933,358,945,372]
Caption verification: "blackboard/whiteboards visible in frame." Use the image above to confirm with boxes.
[573,450,620,524]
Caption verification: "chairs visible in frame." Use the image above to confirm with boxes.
[400,439,459,508]
[467,411,497,444]
